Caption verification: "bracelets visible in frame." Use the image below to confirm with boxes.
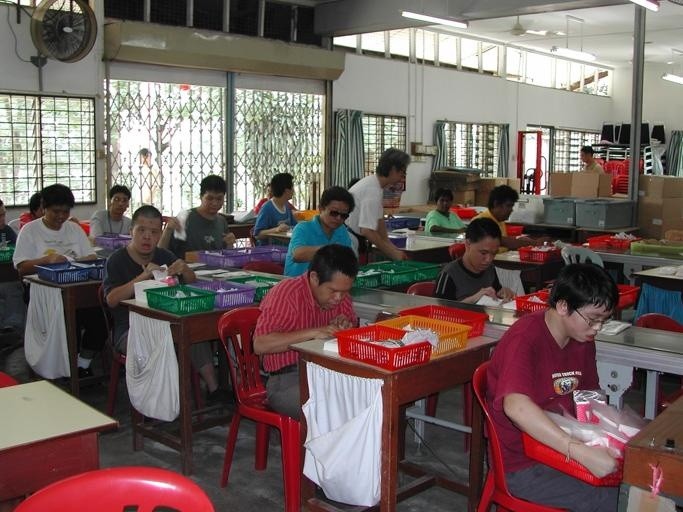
[565,439,578,463]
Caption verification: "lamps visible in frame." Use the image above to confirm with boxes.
[400,0,469,29]
[629,0,660,12]
[549,14,596,63]
[660,48,683,85]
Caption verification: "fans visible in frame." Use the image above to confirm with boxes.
[30,0,98,68]
[500,14,547,36]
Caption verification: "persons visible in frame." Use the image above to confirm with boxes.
[578,144,605,174]
[468,184,519,237]
[284,186,355,278]
[130,148,161,208]
[1,171,296,410]
[482,262,622,512]
[423,185,467,232]
[342,147,410,263]
[431,216,515,306]
[250,242,373,512]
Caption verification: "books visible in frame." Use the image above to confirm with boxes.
[598,320,632,336]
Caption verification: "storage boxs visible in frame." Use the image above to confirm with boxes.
[542,170,683,241]
[431,164,523,209]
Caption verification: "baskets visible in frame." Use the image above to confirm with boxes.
[142,274,284,316]
[387,233,408,248]
[294,207,320,223]
[519,427,628,488]
[513,284,641,313]
[96,231,135,249]
[0,244,17,262]
[199,245,288,268]
[505,224,524,237]
[449,207,477,219]
[349,257,443,288]
[333,304,489,372]
[384,213,420,230]
[586,236,683,259]
[33,256,107,284]
[517,245,562,263]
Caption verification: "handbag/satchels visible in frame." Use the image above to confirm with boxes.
[343,221,372,256]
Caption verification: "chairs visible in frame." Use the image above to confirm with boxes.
[591,119,668,194]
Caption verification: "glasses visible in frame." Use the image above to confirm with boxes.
[321,204,350,221]
[566,300,615,328]
[111,196,130,203]
